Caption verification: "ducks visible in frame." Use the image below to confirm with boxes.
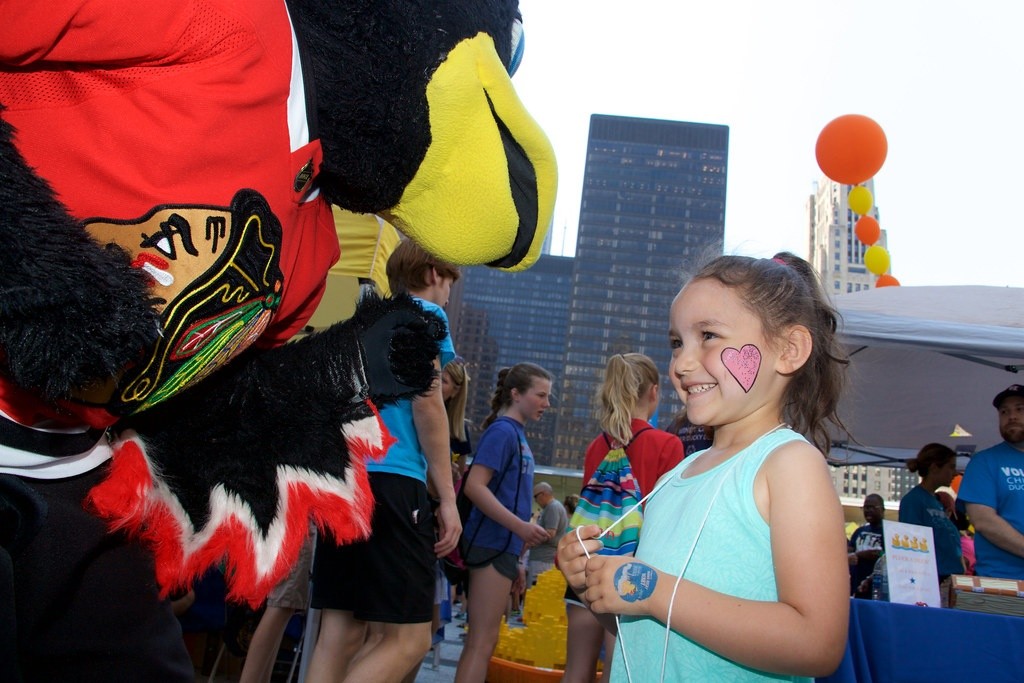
[892,533,928,552]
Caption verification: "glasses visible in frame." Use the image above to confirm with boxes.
[534,491,542,498]
[863,505,880,509]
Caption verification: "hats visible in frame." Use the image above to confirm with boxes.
[992,384,1024,409]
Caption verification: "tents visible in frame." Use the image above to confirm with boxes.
[288,203,401,683]
[820,286,1024,470]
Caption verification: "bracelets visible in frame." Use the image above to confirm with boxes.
[865,576,872,583]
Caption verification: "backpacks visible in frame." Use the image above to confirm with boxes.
[438,419,523,586]
[554,427,655,570]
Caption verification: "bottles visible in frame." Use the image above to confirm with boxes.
[872,552,884,601]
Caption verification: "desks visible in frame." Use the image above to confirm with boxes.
[814,599,1024,683]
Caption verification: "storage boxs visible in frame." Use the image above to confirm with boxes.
[949,574,1024,617]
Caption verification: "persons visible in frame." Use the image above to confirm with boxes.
[441,451,581,639]
[438,356,553,683]
[157,583,195,617]
[846,383,1024,603]
[562,352,714,683]
[556,251,868,683]
[239,239,462,683]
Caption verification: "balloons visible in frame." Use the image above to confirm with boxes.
[816,114,901,288]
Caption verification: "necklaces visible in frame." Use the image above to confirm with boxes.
[576,423,789,683]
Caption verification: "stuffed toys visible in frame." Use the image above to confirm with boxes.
[0,0,557,683]
